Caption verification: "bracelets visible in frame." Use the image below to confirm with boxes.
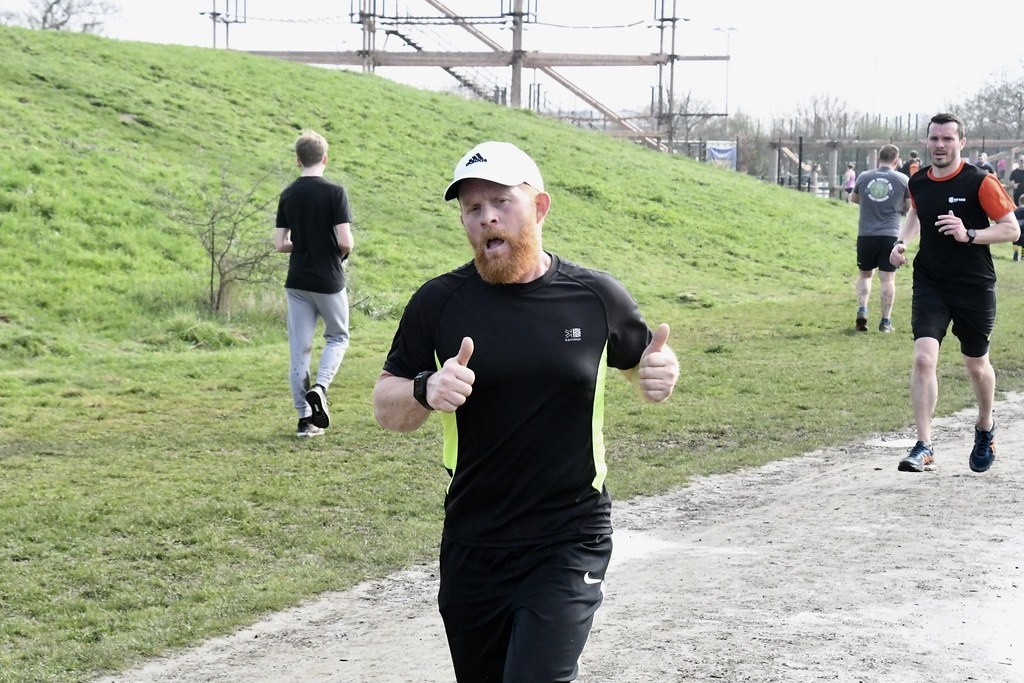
[893,240,903,246]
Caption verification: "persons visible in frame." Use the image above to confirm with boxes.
[896,149,926,216]
[974,152,998,177]
[274,132,355,435]
[1012,194,1024,261]
[843,162,856,203]
[851,144,912,333]
[997,156,1006,179]
[889,113,1021,474]
[1009,154,1024,206]
[370,142,680,683]
[810,164,821,197]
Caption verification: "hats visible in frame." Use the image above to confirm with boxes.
[443,141,544,202]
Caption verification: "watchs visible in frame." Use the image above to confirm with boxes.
[413,370,436,412]
[967,229,977,244]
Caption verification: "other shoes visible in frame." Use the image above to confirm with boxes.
[1014,251,1018,260]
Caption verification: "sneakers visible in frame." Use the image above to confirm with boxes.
[879,322,896,333]
[306,387,333,428]
[857,311,868,330]
[898,441,936,472]
[969,419,995,472]
[297,422,325,437]
